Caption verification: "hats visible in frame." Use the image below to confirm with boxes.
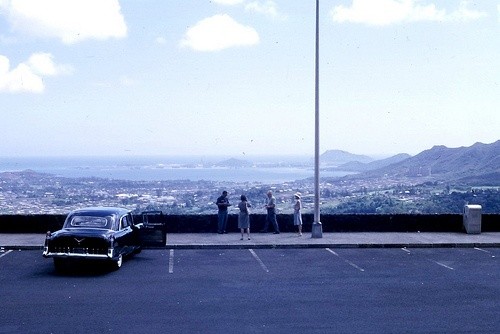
[294,192,302,199]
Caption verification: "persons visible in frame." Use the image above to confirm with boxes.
[216,191,232,234]
[292,192,303,237]
[238,195,251,240]
[260,189,280,234]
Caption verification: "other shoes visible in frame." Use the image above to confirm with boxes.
[260,229,267,233]
[248,238,250,240]
[218,230,227,234]
[240,238,243,240]
[273,231,280,234]
[297,233,303,237]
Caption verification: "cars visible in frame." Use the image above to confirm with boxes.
[42,198,167,273]
